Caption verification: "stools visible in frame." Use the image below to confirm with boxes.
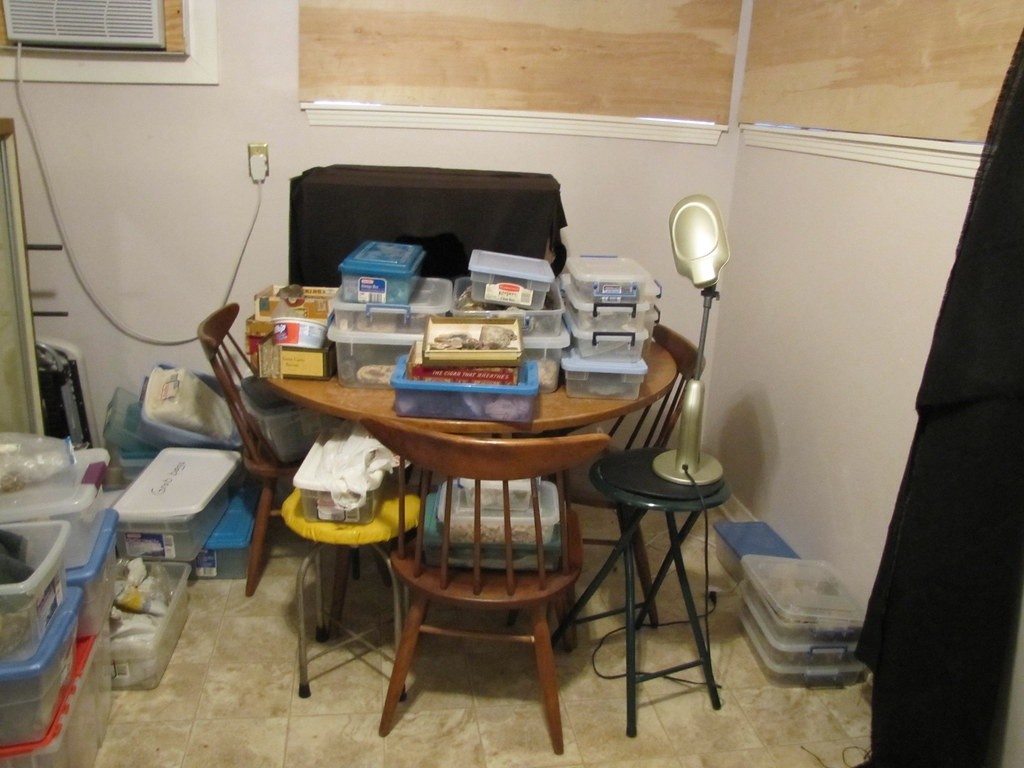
[279,486,425,702]
[551,447,732,738]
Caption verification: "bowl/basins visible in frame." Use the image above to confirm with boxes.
[272,317,327,350]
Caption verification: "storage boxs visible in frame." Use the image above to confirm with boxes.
[735,605,867,689]
[101,362,345,581]
[0,447,111,571]
[712,522,801,584]
[246,240,663,423]
[740,554,864,644]
[0,519,83,749]
[62,508,119,642]
[111,562,191,692]
[736,582,858,666]
[0,628,114,768]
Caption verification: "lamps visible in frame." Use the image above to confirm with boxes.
[654,193,731,485]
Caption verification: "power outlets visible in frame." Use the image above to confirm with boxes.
[247,142,270,176]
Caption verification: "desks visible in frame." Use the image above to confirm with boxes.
[247,339,679,644]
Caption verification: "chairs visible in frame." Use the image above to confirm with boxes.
[197,302,395,598]
[359,413,613,755]
[505,324,706,631]
